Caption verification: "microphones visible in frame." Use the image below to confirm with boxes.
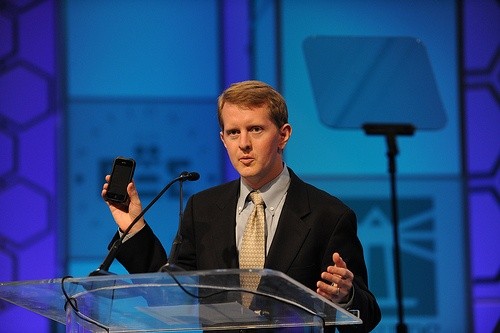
[89,172,200,276]
[158,171,188,273]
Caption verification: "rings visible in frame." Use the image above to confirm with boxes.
[336,287,340,296]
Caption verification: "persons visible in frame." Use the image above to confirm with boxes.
[101,81,382,333]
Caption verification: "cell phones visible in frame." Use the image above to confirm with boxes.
[105,157,136,203]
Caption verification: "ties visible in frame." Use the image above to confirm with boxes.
[238,191,266,309]
